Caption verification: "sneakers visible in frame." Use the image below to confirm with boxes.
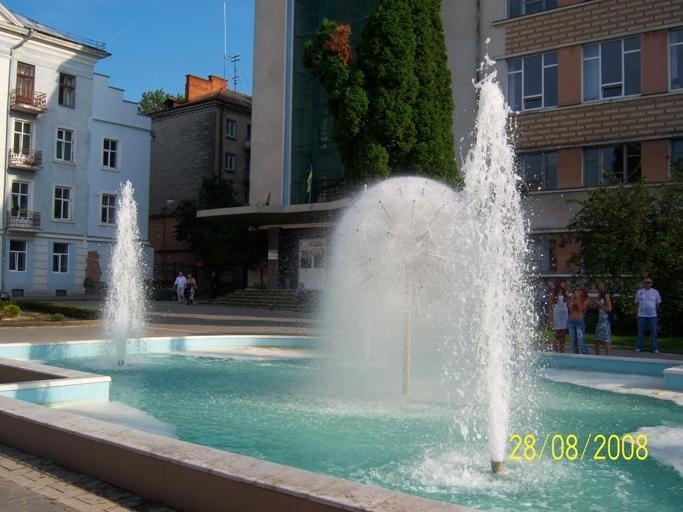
[634,349,660,354]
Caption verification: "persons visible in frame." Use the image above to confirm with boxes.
[545,278,571,352]
[632,275,663,355]
[184,273,199,305]
[592,280,613,356]
[173,271,187,304]
[567,284,590,354]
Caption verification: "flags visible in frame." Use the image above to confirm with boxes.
[305,165,313,193]
